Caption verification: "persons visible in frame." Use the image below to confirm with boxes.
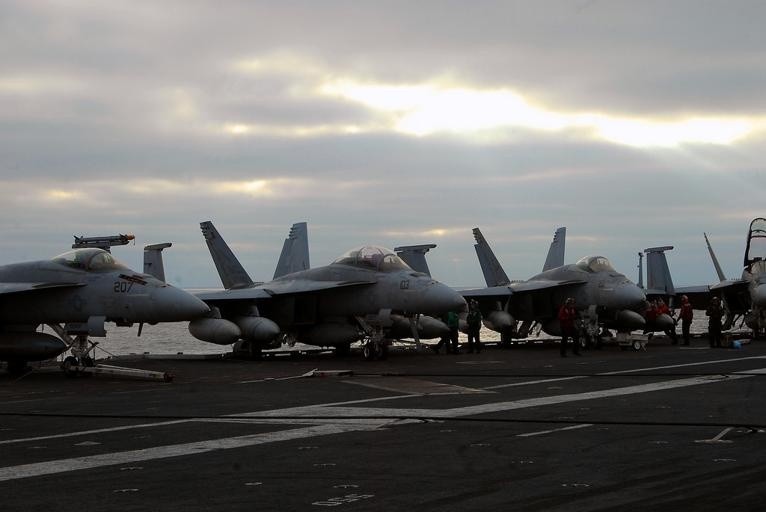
[676,295,693,346]
[446,310,464,354]
[466,299,481,355]
[558,298,583,358]
[706,297,724,348]
[643,295,680,345]
